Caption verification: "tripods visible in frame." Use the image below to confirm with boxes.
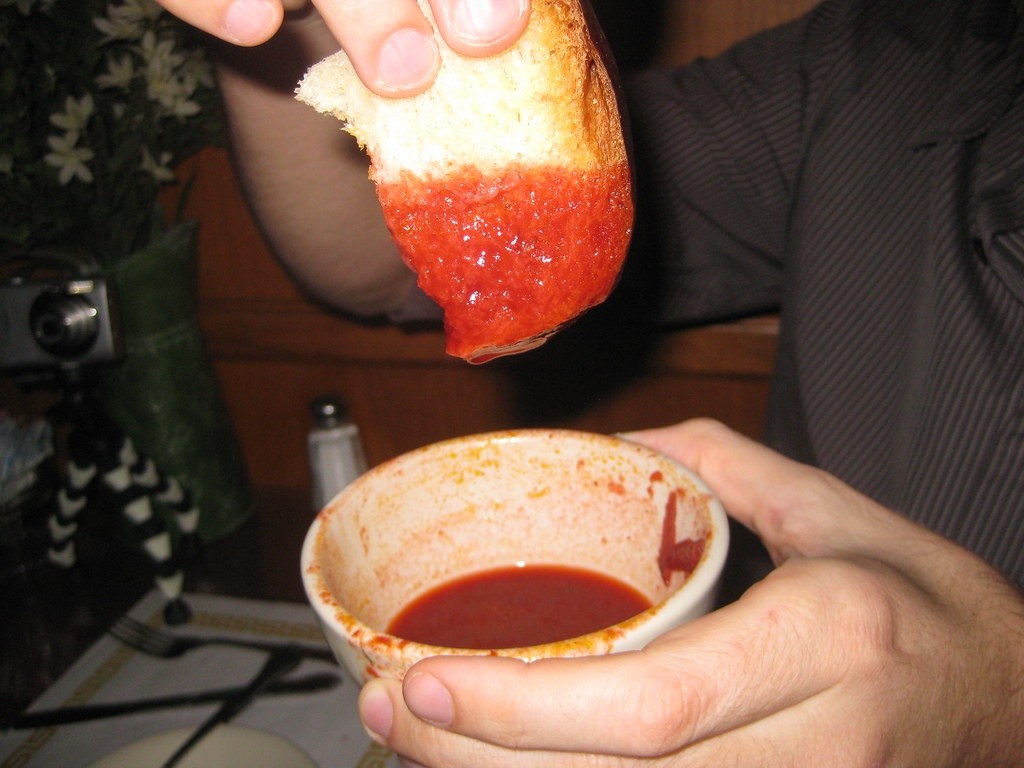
[45,378,205,627]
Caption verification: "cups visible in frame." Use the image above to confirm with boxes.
[299,430,730,690]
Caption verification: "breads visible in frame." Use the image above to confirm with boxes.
[295,0,632,361]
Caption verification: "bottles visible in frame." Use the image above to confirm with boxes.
[308,393,367,515]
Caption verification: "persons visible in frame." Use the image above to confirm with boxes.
[151,0,1024,767]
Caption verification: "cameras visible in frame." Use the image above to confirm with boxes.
[0,273,128,375]
